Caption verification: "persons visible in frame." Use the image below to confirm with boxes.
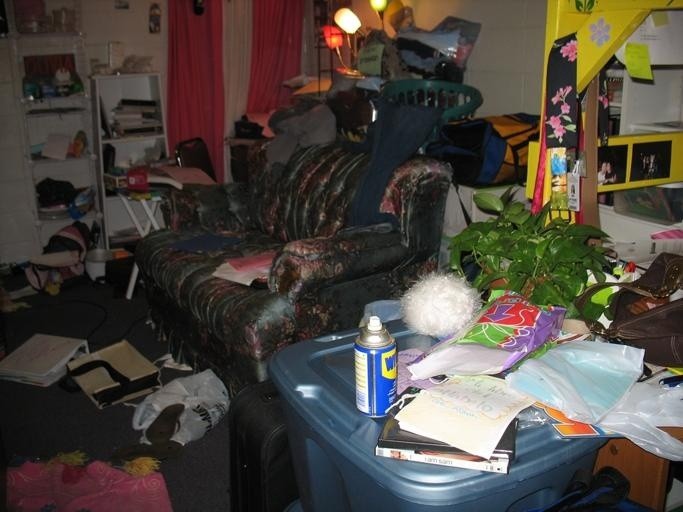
[598,163,617,185]
[642,155,657,178]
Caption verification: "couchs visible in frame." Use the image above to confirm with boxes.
[134,139,453,396]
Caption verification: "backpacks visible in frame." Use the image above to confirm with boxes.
[424,112,541,186]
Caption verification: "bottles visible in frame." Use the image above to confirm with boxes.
[353,315,397,418]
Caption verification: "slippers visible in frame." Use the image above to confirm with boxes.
[99,438,153,468]
[146,404,184,447]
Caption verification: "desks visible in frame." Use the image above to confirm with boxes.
[114,167,216,300]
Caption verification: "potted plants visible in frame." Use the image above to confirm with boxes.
[447,185,614,319]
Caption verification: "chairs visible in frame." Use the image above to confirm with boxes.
[381,78,483,153]
[175,137,217,188]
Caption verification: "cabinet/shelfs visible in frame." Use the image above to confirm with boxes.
[11,32,105,256]
[314,1,353,99]
[91,72,169,250]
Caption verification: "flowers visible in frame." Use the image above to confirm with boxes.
[590,19,610,46]
[545,86,576,143]
[559,40,576,62]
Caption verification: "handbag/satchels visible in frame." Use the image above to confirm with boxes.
[227,378,299,512]
[24,264,52,290]
[522,466,655,511]
[58,339,161,410]
[233,115,262,138]
[92,256,141,290]
[574,252,683,368]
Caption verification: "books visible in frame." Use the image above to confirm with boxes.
[109,99,163,134]
[374,386,518,473]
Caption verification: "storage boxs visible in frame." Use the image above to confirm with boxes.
[267,319,608,512]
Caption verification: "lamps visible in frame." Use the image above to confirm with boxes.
[321,0,388,72]
